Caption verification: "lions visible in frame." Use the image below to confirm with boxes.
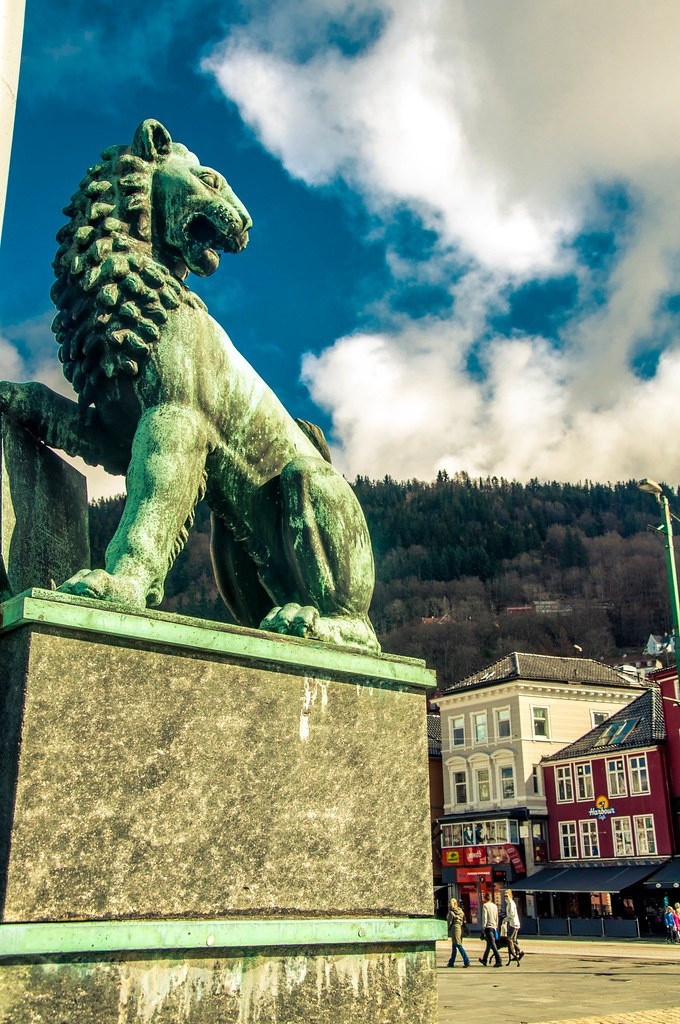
[0,115,390,657]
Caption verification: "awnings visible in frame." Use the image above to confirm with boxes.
[498,854,680,893]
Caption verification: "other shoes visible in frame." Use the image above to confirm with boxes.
[463,963,470,967]
[447,963,455,966]
[518,951,524,960]
[493,962,502,967]
[479,957,487,966]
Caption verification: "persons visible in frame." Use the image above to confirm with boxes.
[478,892,503,967]
[446,898,471,968]
[664,902,680,944]
[503,888,525,960]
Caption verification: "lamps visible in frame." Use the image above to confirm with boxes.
[591,832,606,838]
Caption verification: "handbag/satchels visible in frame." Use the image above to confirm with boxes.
[493,929,498,940]
[480,932,486,940]
[500,921,508,937]
